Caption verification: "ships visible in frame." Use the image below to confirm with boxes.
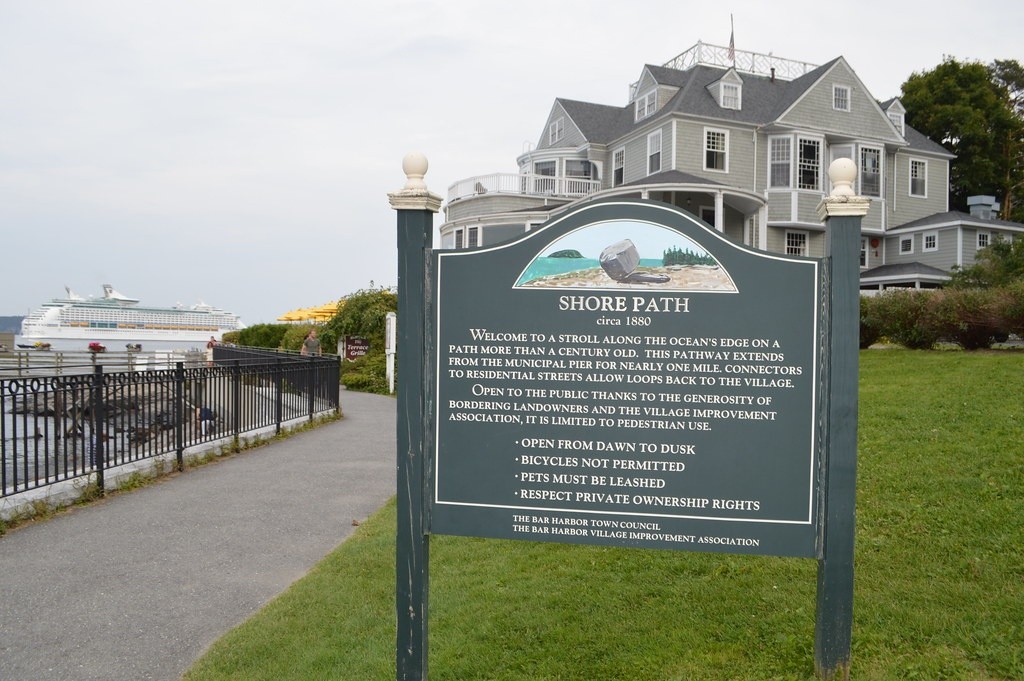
[13,285,246,375]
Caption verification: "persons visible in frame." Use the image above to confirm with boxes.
[224,338,237,348]
[300,330,322,357]
[195,406,216,438]
[207,336,217,366]
